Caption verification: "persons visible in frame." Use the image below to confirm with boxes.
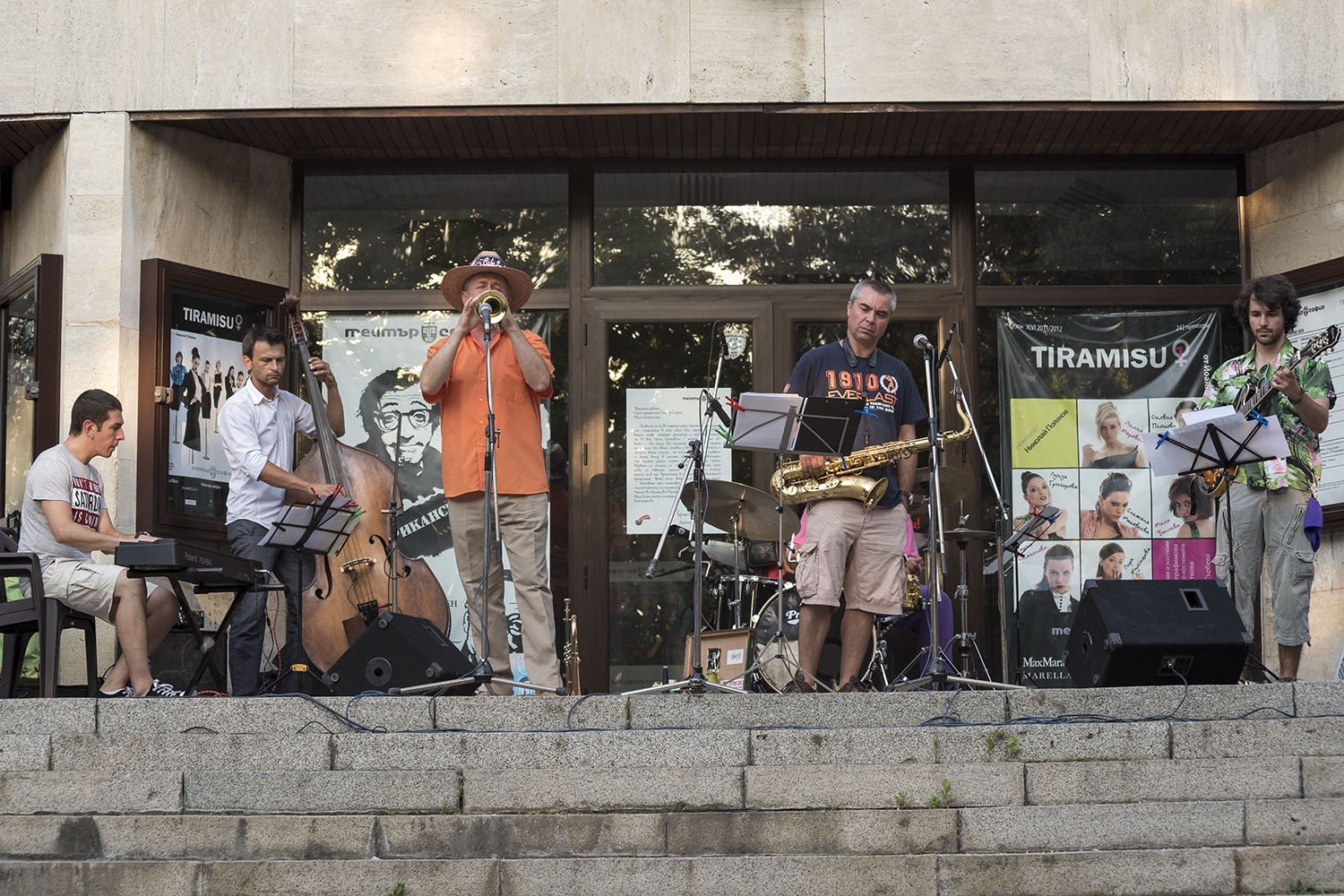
[216,326,345,697]
[782,280,972,693]
[17,387,199,698]
[420,251,565,698]
[171,347,244,451]
[1199,272,1337,681]
[1016,402,1215,612]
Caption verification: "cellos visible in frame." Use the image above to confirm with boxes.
[278,291,452,674]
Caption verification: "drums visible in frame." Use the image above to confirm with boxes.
[752,583,877,694]
[712,574,785,630]
[687,539,754,613]
[902,573,922,611]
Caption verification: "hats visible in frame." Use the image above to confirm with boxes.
[442,251,534,312]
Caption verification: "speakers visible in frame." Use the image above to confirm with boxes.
[317,610,482,696]
[118,632,228,697]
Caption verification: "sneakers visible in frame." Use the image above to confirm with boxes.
[833,675,870,692]
[94,679,186,698]
[780,669,818,693]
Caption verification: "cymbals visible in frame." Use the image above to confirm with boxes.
[680,480,801,542]
[907,466,971,519]
[937,528,997,543]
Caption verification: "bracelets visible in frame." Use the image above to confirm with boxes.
[1288,387,1306,405]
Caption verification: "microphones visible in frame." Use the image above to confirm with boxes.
[704,389,731,428]
[478,303,492,334]
[717,321,729,356]
[913,334,934,350]
[936,323,956,368]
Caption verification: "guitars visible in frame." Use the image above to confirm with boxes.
[1193,324,1341,497]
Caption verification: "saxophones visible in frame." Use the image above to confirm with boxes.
[769,380,973,512]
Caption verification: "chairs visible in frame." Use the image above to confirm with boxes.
[0,530,98,699]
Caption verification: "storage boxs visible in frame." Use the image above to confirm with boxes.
[682,628,758,694]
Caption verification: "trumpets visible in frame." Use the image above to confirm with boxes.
[474,290,508,333]
[562,598,581,696]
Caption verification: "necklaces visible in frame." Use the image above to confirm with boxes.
[1258,348,1277,366]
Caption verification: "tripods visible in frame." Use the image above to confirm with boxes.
[256,493,358,697]
[621,349,836,696]
[884,352,1062,693]
[388,335,567,697]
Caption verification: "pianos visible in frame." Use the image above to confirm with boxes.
[114,539,287,696]
